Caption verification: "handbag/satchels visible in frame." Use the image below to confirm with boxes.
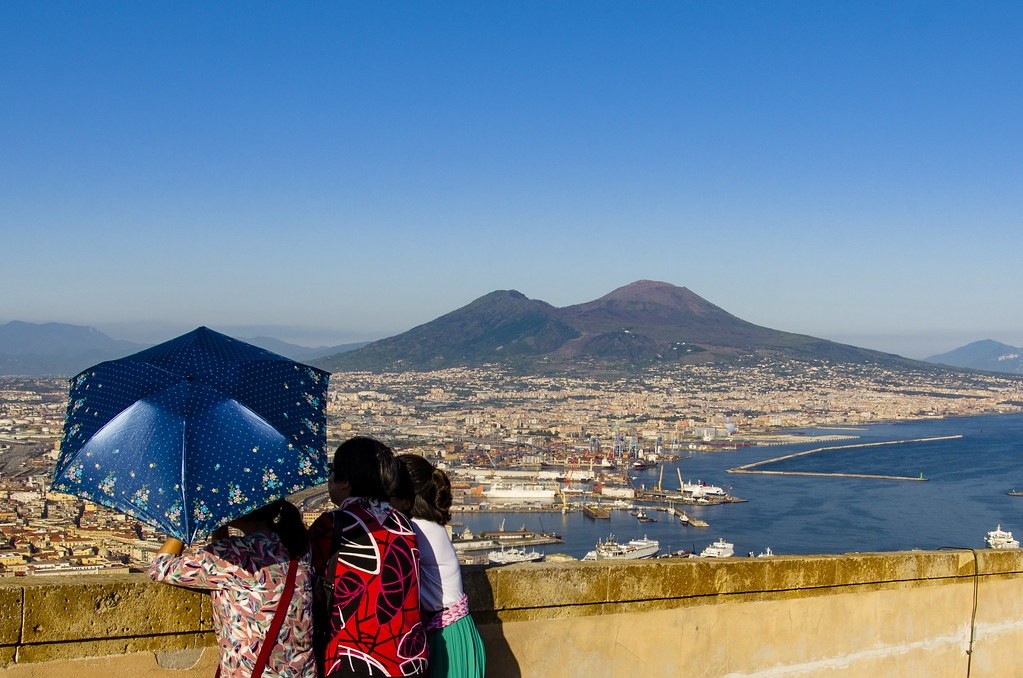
[311,510,345,658]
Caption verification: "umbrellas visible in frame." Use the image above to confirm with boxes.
[49,325,334,550]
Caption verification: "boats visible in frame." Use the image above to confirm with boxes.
[600,457,617,471]
[667,507,676,515]
[679,514,689,525]
[756,546,775,557]
[479,481,556,499]
[677,478,727,496]
[560,487,585,496]
[580,532,660,561]
[699,537,735,559]
[488,542,547,567]
[984,524,1020,549]
[632,457,658,469]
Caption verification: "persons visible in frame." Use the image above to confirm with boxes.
[392,453,487,678]
[149,496,317,678]
[307,435,434,678]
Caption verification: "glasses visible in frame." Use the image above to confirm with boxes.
[328,462,335,473]
